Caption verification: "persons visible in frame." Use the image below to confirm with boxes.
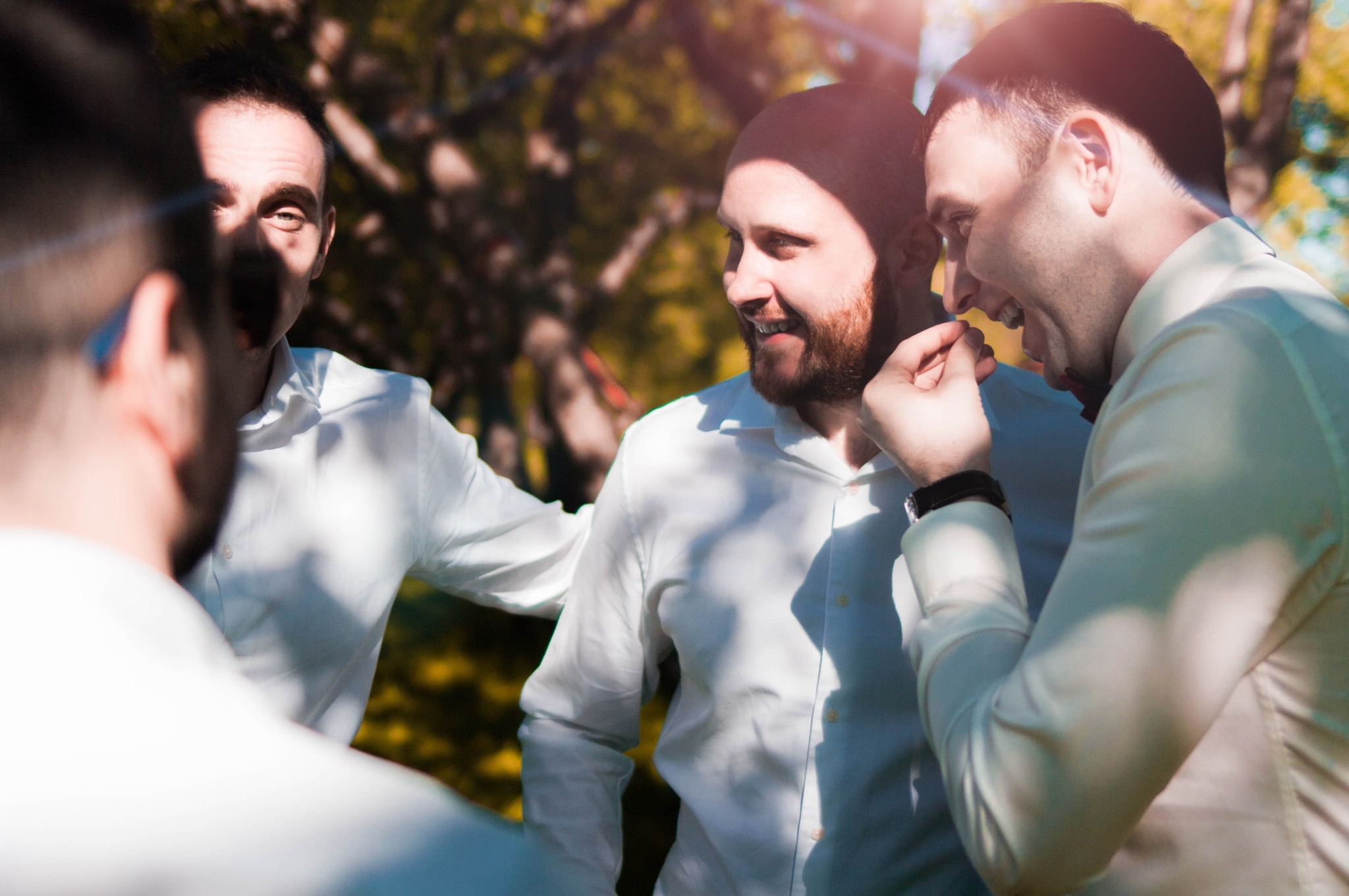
[0,0,623,894]
[141,40,601,783]
[863,3,1347,893]
[482,76,1135,896]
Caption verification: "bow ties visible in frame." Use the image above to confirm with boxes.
[1065,369,1104,421]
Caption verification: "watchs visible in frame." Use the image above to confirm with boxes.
[903,470,1017,530]
